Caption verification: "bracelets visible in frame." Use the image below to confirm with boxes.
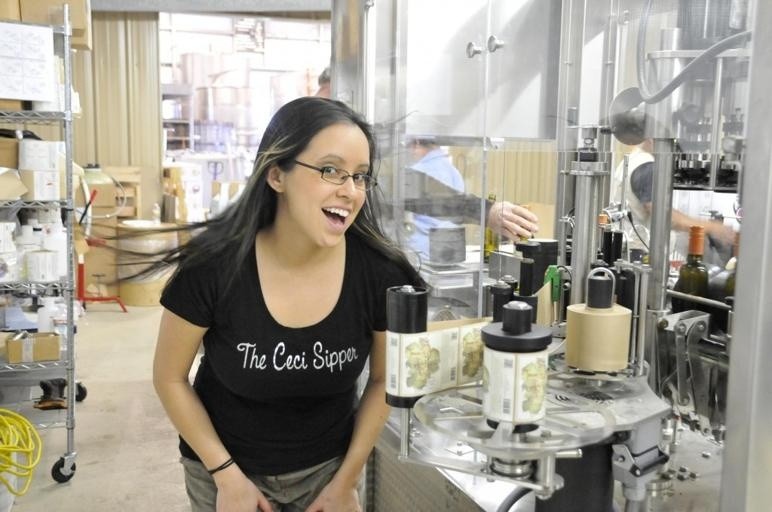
[209,457,234,475]
[208,460,234,473]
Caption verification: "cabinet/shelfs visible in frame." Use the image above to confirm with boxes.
[0,0,78,482]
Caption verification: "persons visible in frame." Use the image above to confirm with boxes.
[96,97,429,512]
[404,132,465,263]
[370,160,541,242]
[612,139,741,279]
[315,68,331,99]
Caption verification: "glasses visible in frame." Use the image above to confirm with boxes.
[290,160,377,190]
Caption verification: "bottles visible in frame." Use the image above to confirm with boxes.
[678,225,709,311]
